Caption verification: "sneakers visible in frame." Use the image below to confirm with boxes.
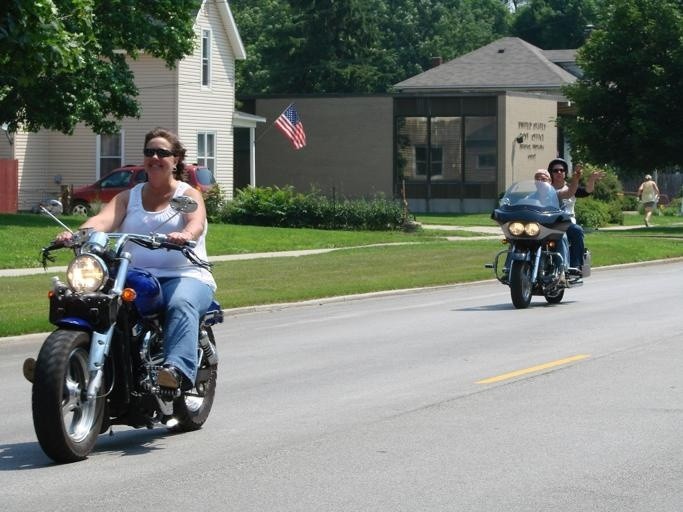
[559,265,584,280]
[152,365,182,403]
[21,357,38,383]
[500,273,508,283]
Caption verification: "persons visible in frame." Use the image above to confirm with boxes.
[637,174,660,227]
[547,158,607,223]
[522,164,584,276]
[20,127,216,403]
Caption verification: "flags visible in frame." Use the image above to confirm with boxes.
[274,105,308,152]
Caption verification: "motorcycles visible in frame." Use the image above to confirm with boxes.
[32,196,223,462]
[485,182,592,309]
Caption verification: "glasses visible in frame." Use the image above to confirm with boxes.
[141,147,175,159]
[551,168,565,174]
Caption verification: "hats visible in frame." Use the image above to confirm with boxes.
[548,158,568,176]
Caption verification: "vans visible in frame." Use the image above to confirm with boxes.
[65,163,218,216]
